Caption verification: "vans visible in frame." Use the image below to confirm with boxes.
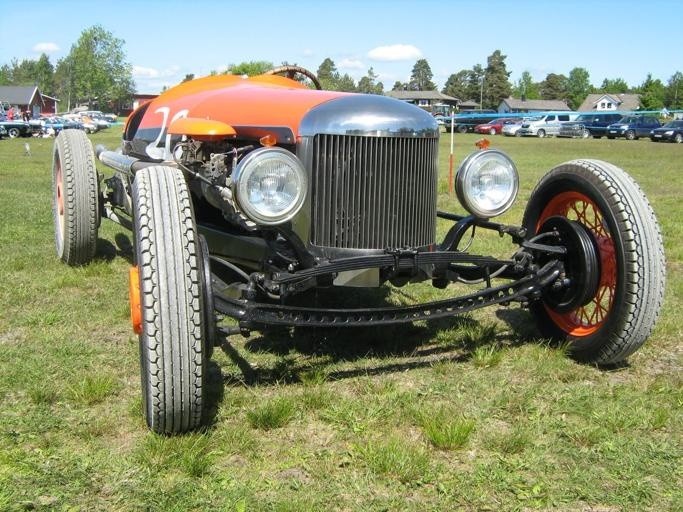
[447,112,579,138]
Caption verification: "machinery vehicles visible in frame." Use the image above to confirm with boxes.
[52,69,666,433]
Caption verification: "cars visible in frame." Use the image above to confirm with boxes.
[650,119,683,142]
[0,109,117,138]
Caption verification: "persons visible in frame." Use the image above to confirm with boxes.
[7,105,15,120]
[23,108,30,122]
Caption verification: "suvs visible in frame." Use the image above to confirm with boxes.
[562,113,622,139]
[606,116,660,140]
